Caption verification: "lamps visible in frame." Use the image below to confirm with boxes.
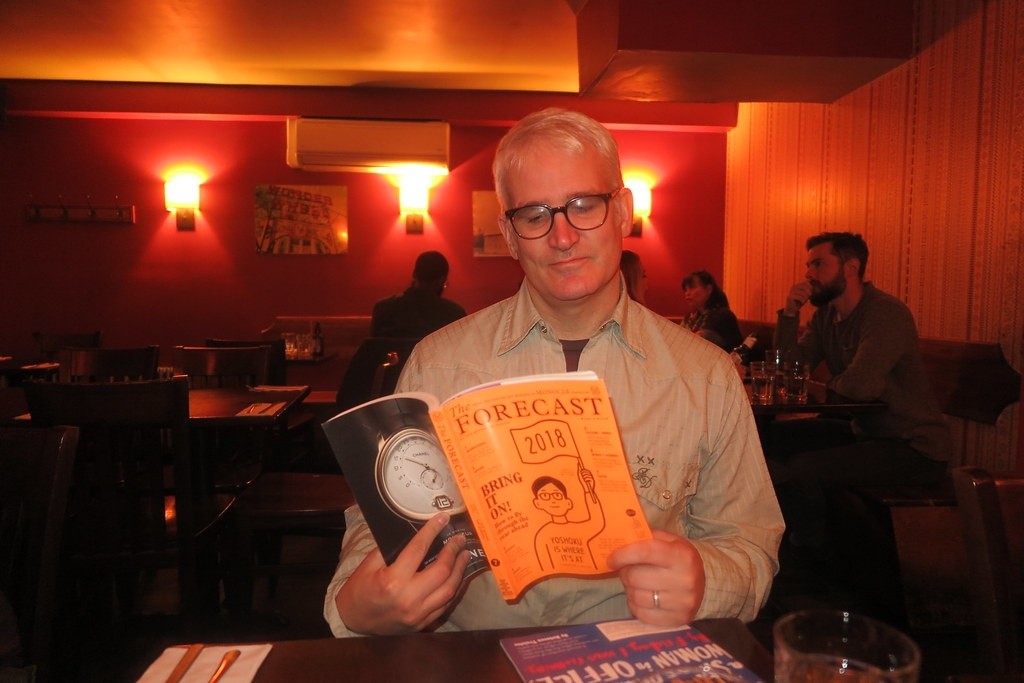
[628,174,652,237]
[163,174,201,230]
[398,168,431,235]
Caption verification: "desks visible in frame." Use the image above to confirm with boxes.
[737,382,888,438]
[119,619,774,683]
[176,386,311,474]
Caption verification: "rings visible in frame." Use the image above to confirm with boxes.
[653,590,661,609]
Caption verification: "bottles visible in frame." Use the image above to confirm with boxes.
[311,323,324,357]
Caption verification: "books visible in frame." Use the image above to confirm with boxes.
[320,371,656,605]
[499,620,767,683]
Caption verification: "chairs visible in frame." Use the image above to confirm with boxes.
[952,468,1023,674]
[883,339,1020,635]
[0,329,384,683]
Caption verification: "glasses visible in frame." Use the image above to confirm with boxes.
[505,189,621,240]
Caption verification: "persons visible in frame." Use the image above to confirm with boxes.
[319,106,787,639]
[679,269,744,355]
[335,251,467,411]
[766,231,953,596]
[620,250,648,303]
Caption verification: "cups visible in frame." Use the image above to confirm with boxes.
[750,361,777,405]
[781,360,810,406]
[766,348,791,405]
[773,608,920,683]
[280,333,297,360]
[296,334,312,359]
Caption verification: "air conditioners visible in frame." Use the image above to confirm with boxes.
[295,116,451,175]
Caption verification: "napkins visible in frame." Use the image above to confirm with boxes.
[136,642,271,683]
